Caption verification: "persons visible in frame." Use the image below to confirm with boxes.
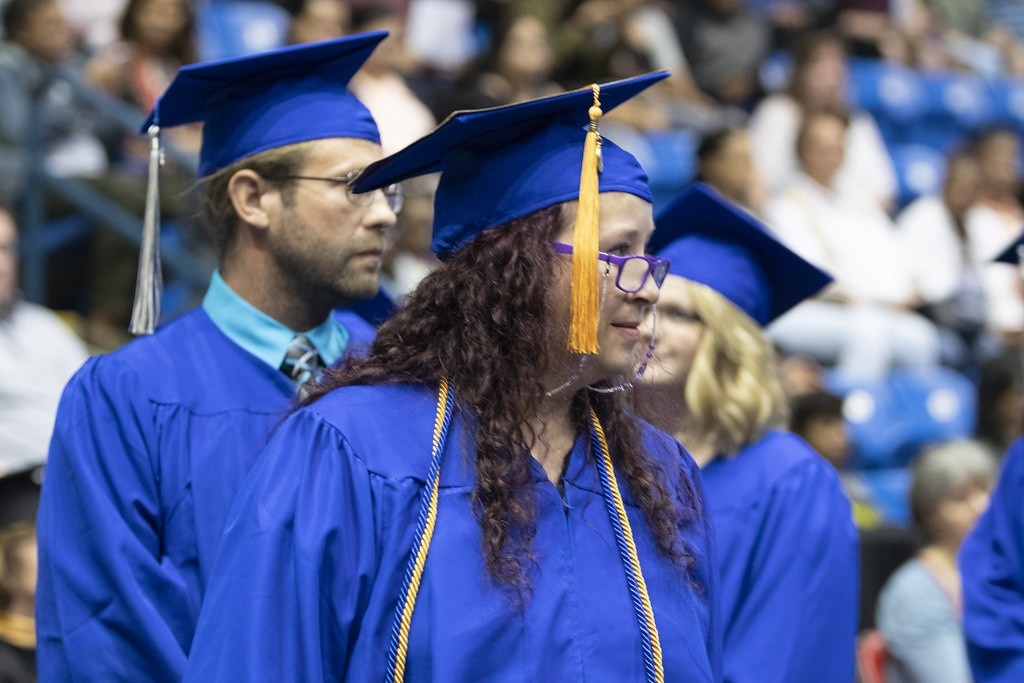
[0,0,1024,501]
[33,29,404,682]
[188,67,723,683]
[879,435,994,683]
[633,184,858,683]
[960,441,1024,683]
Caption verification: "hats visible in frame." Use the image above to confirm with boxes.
[353,68,674,257]
[644,181,836,329]
[137,29,386,179]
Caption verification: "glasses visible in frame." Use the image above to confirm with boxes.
[552,240,672,294]
[264,169,404,214]
